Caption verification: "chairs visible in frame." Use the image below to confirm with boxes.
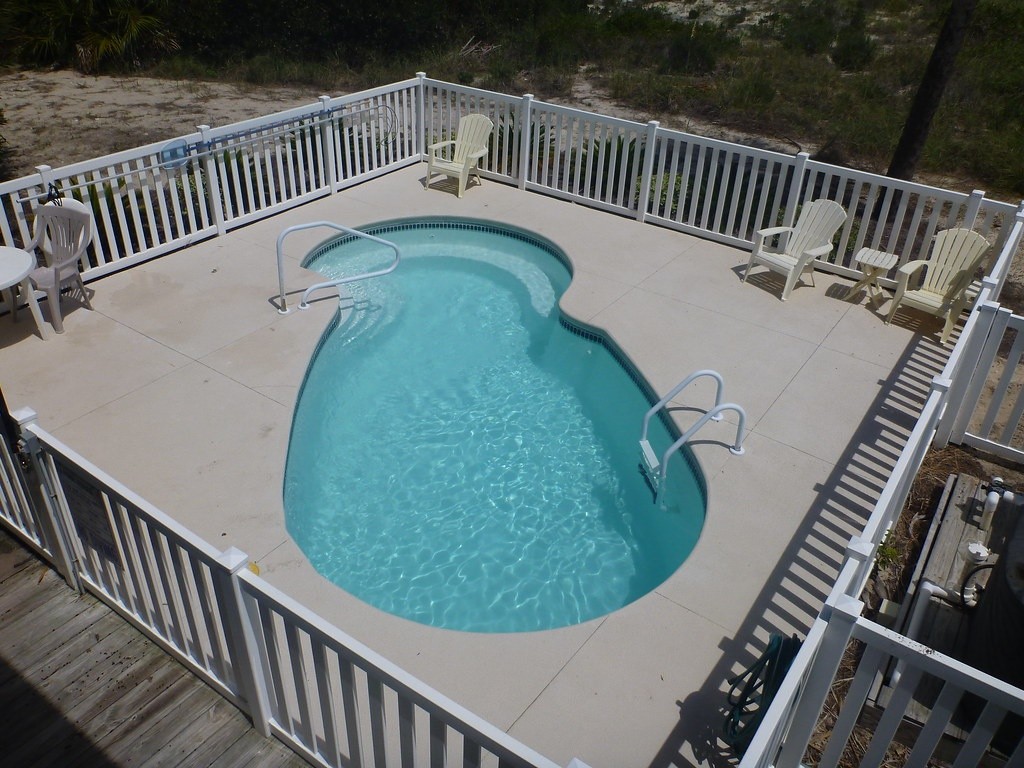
[11,204,94,334]
[424,113,494,198]
[742,199,848,303]
[885,228,992,345]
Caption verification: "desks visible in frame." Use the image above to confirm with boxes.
[843,246,899,310]
[0,246,51,341]
